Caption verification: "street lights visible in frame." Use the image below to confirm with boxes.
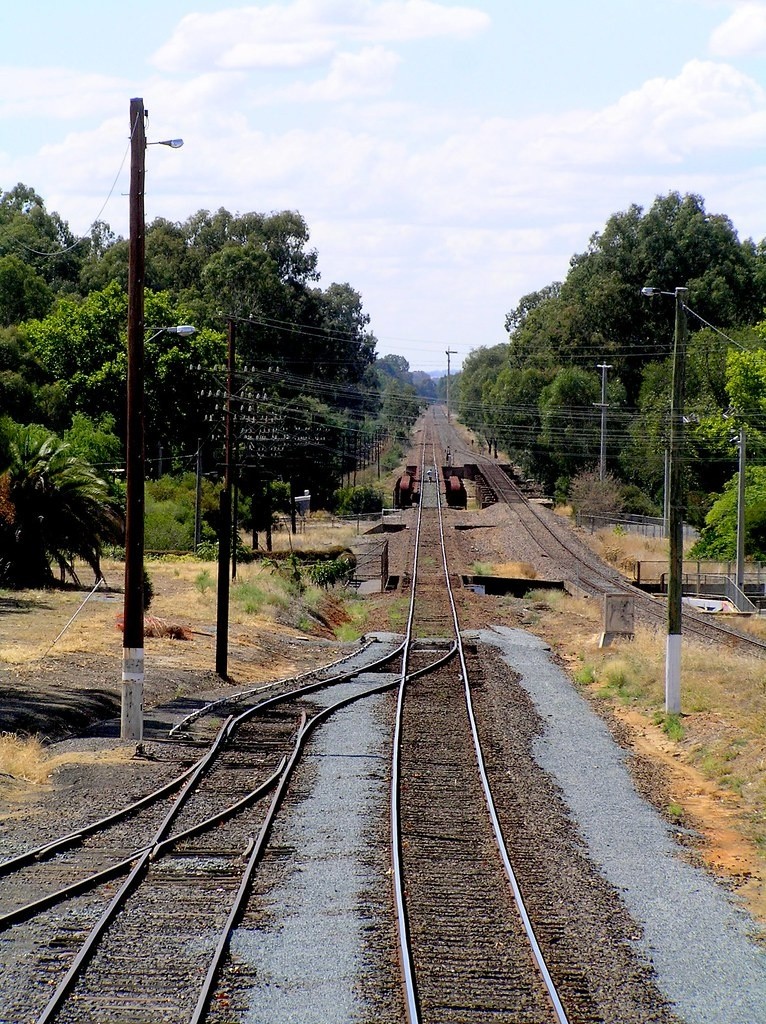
[121,97,197,740]
[641,285,691,718]
